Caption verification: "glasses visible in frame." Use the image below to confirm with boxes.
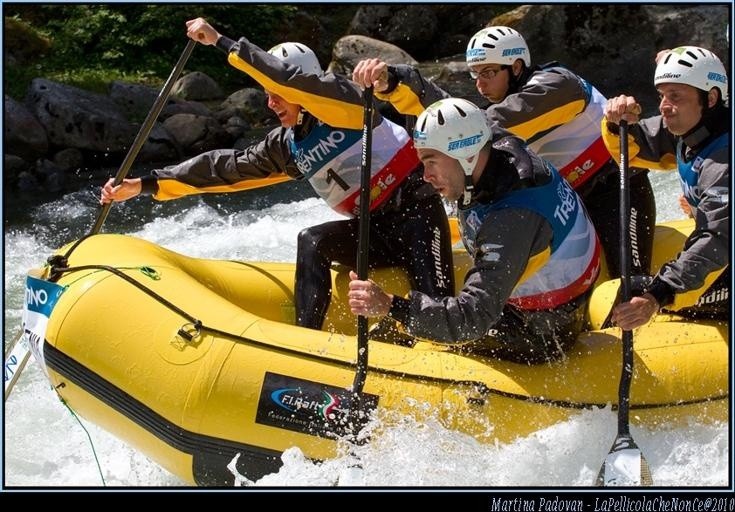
[469,68,501,80]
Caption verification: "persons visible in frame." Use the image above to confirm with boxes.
[354,23,659,280]
[346,58,604,367]
[95,16,460,335]
[599,46,730,336]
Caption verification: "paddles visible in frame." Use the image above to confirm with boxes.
[2,34,205,399]
[596,104,654,486]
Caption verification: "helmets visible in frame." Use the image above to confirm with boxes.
[653,46,729,103]
[413,98,491,175]
[265,42,323,79]
[466,26,531,68]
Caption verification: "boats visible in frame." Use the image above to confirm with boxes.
[22,218,733,487]
